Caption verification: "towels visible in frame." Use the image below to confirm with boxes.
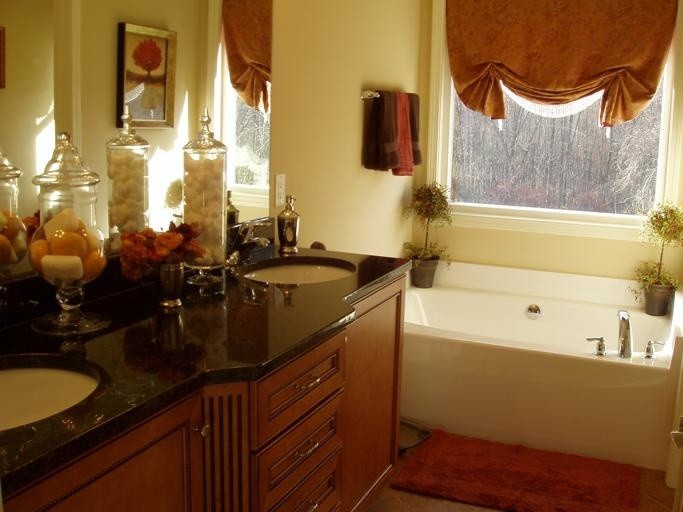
[372,90,422,176]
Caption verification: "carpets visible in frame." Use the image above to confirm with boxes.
[390,431,639,512]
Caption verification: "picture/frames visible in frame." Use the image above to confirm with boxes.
[116,21,178,128]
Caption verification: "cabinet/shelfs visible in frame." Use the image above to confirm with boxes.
[1,271,405,512]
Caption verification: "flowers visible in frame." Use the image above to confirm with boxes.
[120,220,207,283]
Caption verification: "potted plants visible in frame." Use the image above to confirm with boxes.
[627,200,683,315]
[403,180,452,288]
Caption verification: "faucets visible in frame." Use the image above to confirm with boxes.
[616,308,633,359]
[234,216,271,259]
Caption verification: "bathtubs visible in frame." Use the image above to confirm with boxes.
[399,283,682,470]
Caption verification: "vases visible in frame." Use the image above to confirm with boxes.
[159,262,184,308]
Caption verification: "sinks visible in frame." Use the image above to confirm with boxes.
[0,350,100,432]
[243,254,356,284]
